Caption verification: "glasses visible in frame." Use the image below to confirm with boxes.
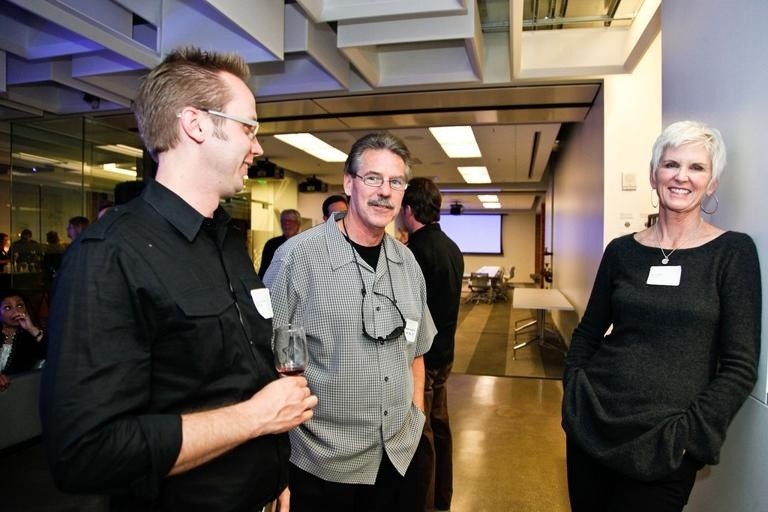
[177,102,261,139]
[360,291,407,347]
[350,171,410,192]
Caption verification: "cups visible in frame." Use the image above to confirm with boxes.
[274,323,309,379]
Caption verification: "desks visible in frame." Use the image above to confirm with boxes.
[510,286,575,370]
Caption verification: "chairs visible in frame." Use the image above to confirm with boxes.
[463,265,516,305]
[2,370,45,459]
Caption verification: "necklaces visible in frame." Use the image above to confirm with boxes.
[654,216,704,265]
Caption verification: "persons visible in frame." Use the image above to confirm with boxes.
[258,209,302,282]
[261,129,438,511]
[402,177,464,510]
[322,195,348,222]
[37,43,321,509]
[560,121,764,512]
[1,201,117,395]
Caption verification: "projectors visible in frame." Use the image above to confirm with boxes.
[247,159,285,180]
[298,177,329,192]
[450,205,463,214]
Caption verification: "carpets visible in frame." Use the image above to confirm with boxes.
[452,295,568,381]
